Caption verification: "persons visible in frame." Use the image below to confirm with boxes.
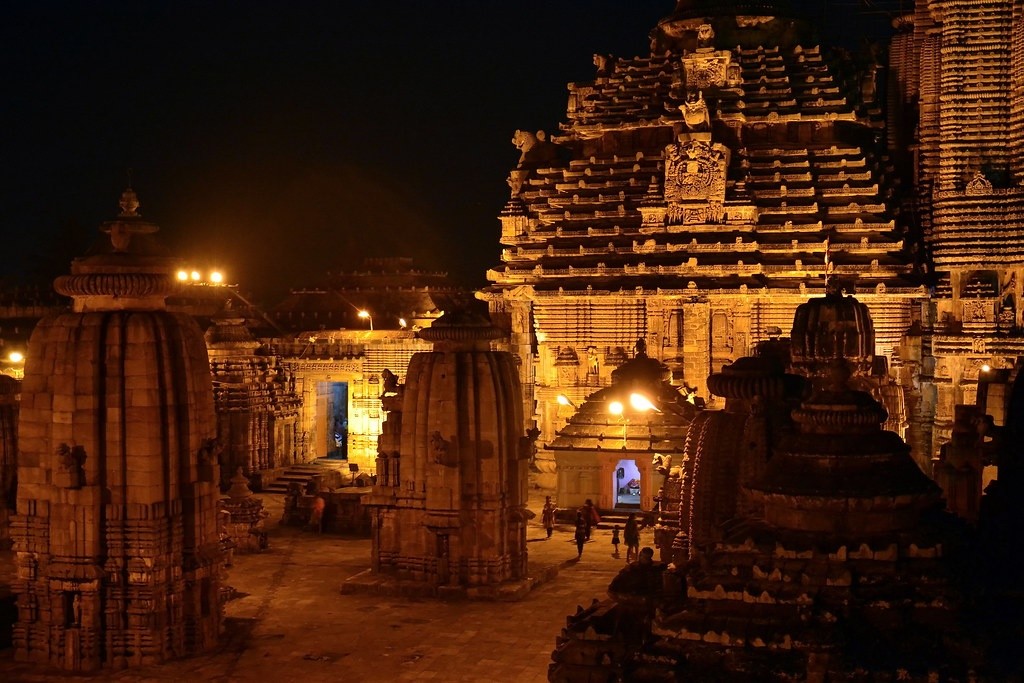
[575,512,587,560]
[612,524,621,557]
[579,498,595,539]
[541,496,558,538]
[622,513,641,564]
